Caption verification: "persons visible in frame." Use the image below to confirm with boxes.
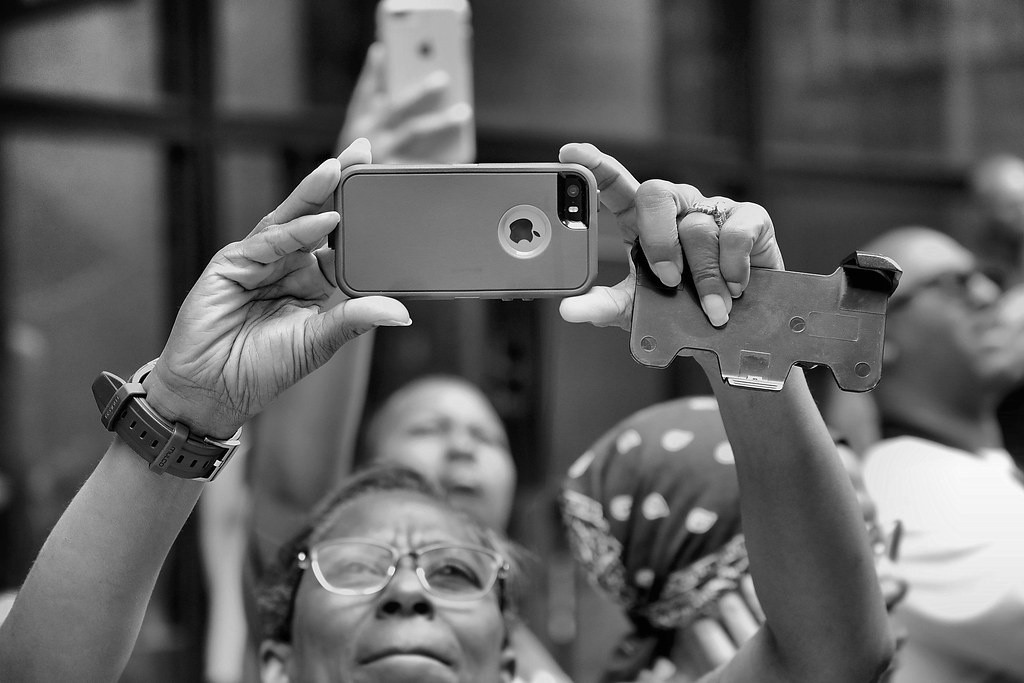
[0,44,1024,683]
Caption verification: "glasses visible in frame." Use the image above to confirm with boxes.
[886,266,1005,317]
[280,538,509,644]
[865,520,901,561]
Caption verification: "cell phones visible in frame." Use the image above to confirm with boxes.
[331,162,601,301]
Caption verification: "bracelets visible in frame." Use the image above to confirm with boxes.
[91,356,244,483]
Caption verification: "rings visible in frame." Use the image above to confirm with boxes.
[682,202,725,230]
